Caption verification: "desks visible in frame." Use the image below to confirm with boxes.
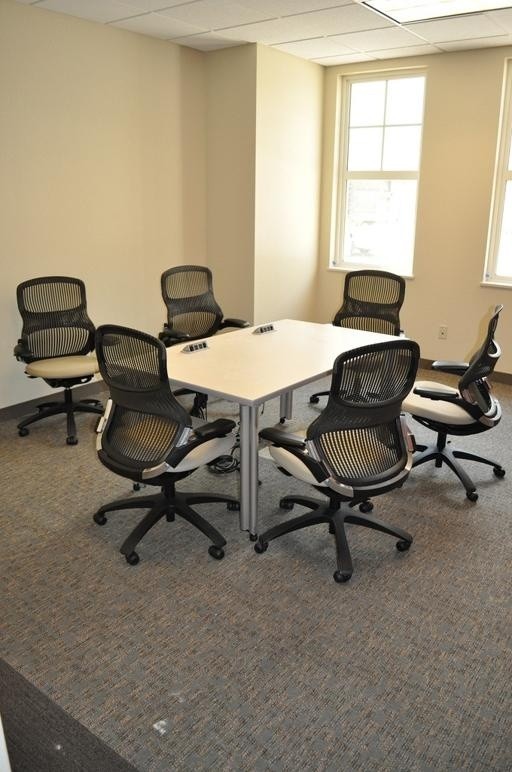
[109,318,412,541]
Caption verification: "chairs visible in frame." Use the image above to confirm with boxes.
[158,265,250,421]
[310,270,406,403]
[13,275,121,445]
[401,305,505,503]
[93,324,240,566]
[254,340,420,583]
